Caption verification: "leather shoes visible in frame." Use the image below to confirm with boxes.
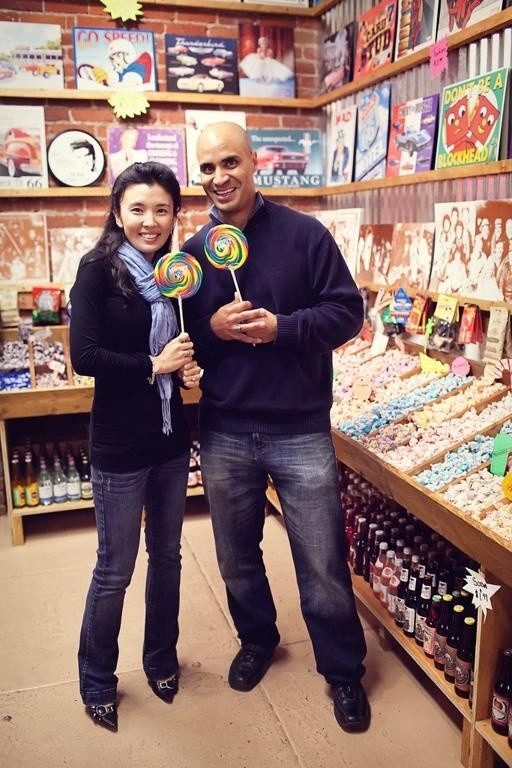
[334,681,371,733]
[149,673,178,704]
[229,643,275,692]
[86,704,118,731]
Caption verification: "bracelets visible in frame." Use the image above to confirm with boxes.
[146,353,160,385]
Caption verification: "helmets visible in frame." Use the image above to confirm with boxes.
[107,39,136,59]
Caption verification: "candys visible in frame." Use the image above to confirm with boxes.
[153,252,202,339]
[321,351,421,407]
[473,502,512,542]
[410,420,512,491]
[334,373,441,432]
[334,338,370,356]
[437,465,504,514]
[376,391,511,474]
[71,368,96,386]
[204,224,249,306]
[332,346,374,372]
[1,339,31,389]
[33,343,65,387]
[353,380,506,473]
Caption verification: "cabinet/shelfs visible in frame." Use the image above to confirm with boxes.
[0,1,512,199]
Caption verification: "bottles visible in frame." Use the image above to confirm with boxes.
[506,687,512,750]
[11,439,91,505]
[331,465,476,710]
[188,440,202,488]
[490,649,508,734]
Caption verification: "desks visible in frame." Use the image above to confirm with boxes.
[0,323,206,547]
[266,385,512,768]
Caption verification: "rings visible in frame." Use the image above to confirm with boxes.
[235,323,243,332]
[191,377,198,384]
[184,349,191,358]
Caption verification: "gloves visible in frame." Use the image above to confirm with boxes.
[92,67,108,83]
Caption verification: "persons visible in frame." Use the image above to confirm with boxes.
[178,122,370,731]
[352,200,512,302]
[69,161,205,732]
[240,36,296,87]
[328,129,352,181]
[111,130,149,180]
